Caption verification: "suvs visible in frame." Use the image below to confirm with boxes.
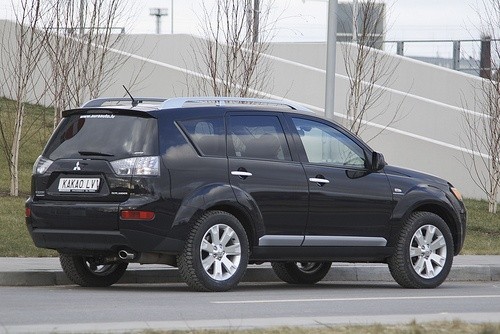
[24,98,467,291]
